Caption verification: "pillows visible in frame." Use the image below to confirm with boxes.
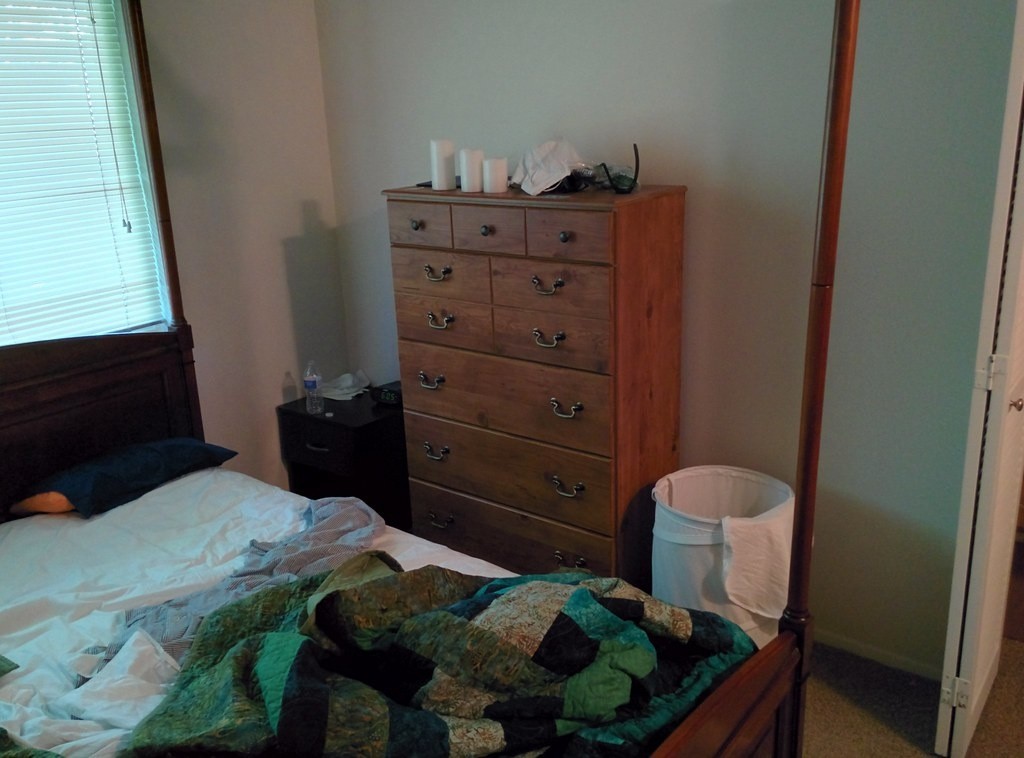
[6,437,240,521]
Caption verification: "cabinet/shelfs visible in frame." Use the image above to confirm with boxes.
[378,183,688,599]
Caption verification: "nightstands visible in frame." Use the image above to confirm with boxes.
[275,384,410,531]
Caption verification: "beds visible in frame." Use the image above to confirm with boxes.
[0,0,861,757]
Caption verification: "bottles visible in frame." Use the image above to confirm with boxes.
[303,361,325,414]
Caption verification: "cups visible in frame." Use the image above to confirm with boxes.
[459,148,484,192]
[430,140,456,190]
[484,157,508,193]
